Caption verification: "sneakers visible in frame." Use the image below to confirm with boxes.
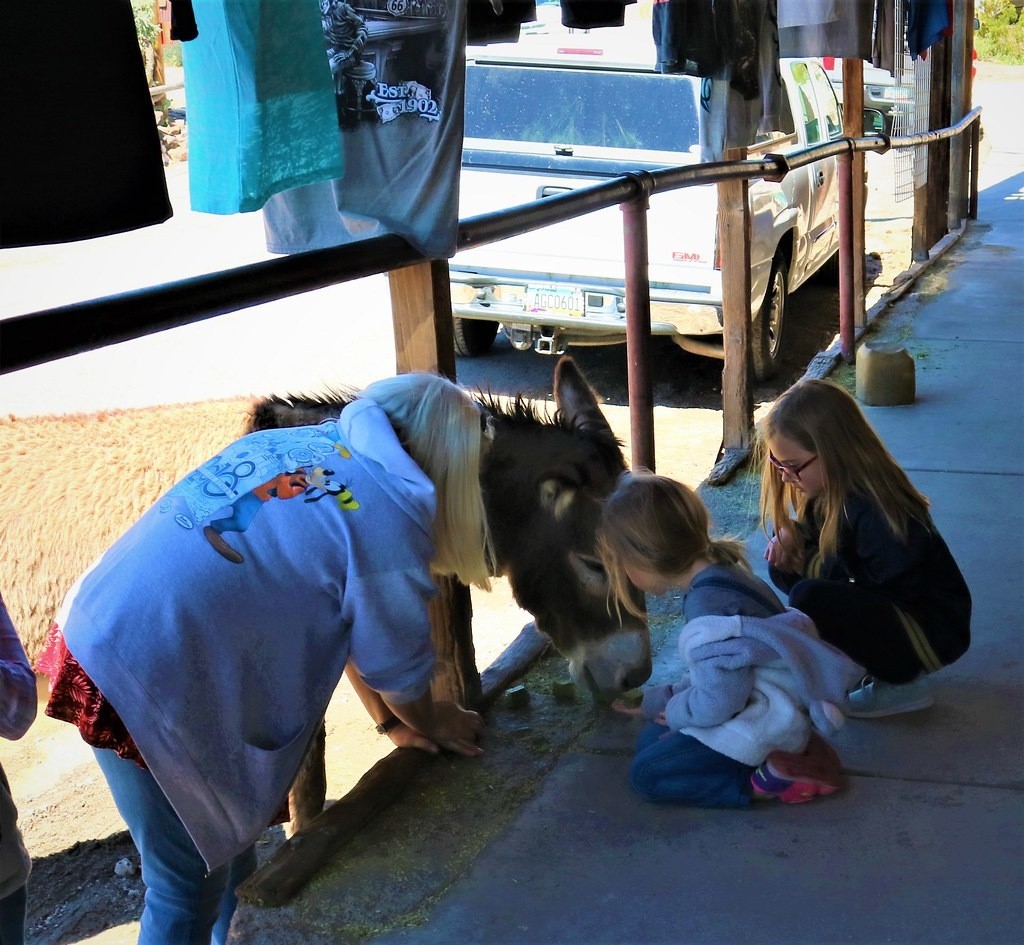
[752,751,841,803]
[845,674,935,717]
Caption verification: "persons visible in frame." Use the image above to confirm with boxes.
[0,591,37,945]
[37,370,494,945]
[598,475,867,809]
[755,377,973,718]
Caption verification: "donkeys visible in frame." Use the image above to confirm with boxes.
[0,355,654,838]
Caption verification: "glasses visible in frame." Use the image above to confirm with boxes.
[768,446,819,483]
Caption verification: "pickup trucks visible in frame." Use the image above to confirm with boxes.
[448,33,887,381]
[819,16,979,132]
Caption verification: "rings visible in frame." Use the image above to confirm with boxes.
[470,719,480,730]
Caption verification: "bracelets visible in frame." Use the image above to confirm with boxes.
[376,716,401,734]
